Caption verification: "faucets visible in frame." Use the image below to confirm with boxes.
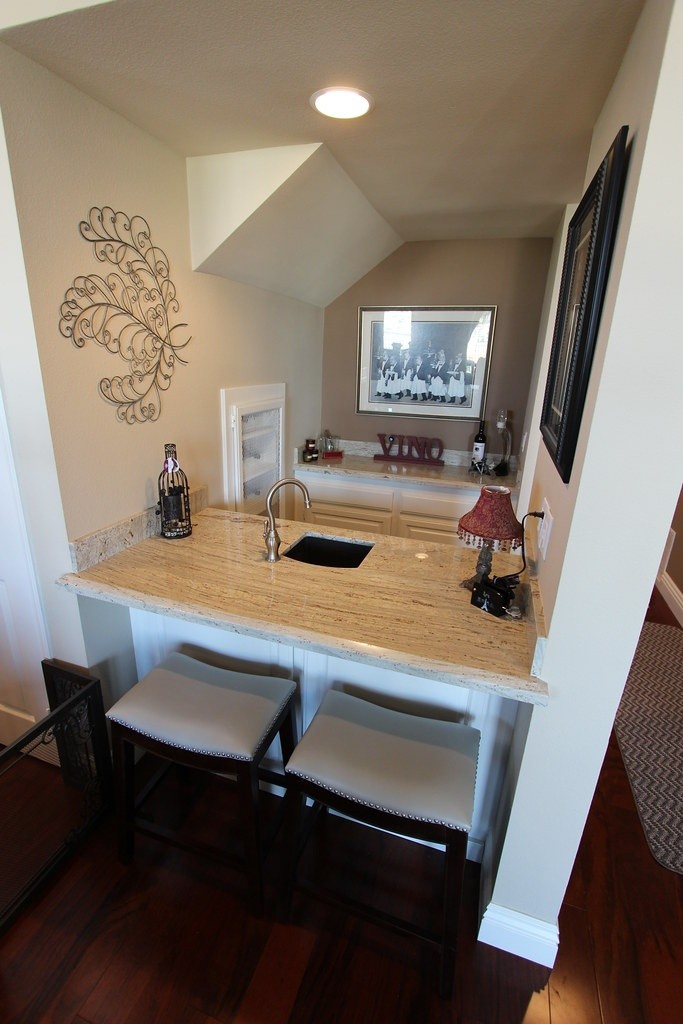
[262,477,312,563]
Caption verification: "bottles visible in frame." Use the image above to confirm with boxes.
[472,421,486,470]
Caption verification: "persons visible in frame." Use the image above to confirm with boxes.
[374,343,467,404]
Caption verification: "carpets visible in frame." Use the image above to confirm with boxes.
[614,621,683,875]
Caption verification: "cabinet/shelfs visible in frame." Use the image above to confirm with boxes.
[293,470,517,552]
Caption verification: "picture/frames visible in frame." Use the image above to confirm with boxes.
[538,124,630,484]
[355,304,498,421]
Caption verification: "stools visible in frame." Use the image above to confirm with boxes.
[101,651,300,916]
[284,687,482,1001]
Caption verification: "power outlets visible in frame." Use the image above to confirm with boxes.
[536,498,550,533]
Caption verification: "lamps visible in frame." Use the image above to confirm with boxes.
[453,484,524,591]
